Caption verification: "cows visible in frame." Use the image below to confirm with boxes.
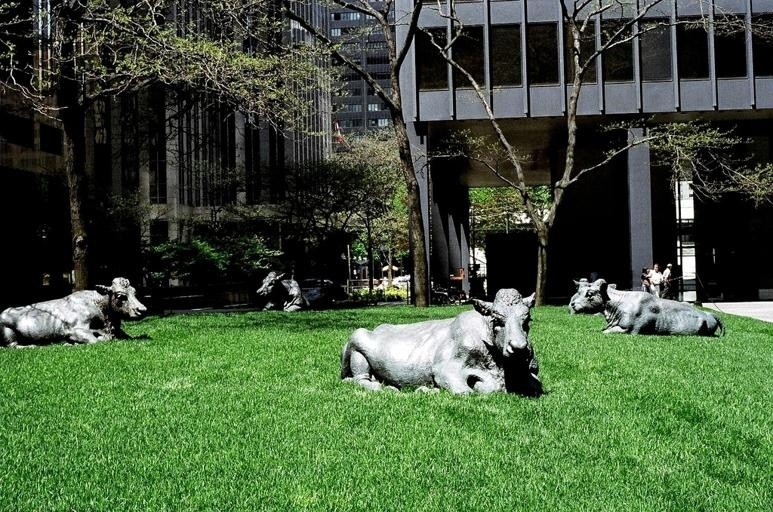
[0,274,149,349]
[570,276,725,340]
[338,286,544,397]
[255,270,353,313]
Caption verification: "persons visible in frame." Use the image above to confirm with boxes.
[661,262,673,300]
[639,266,650,293]
[641,263,664,298]
